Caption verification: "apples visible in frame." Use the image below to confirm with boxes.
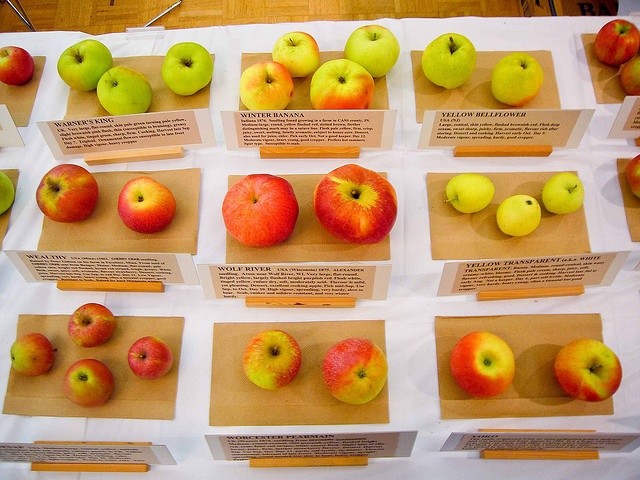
[36,164,99,223]
[68,303,116,349]
[626,154,640,200]
[117,177,176,234]
[238,60,294,111]
[58,38,112,93]
[222,173,300,248]
[0,170,15,216]
[323,337,389,405]
[96,65,152,116]
[491,53,544,108]
[0,46,35,86]
[540,172,585,215]
[344,24,401,78]
[128,335,174,381]
[443,173,496,215]
[620,55,640,96]
[161,41,214,97]
[553,337,623,402]
[313,164,398,244]
[242,330,303,391]
[63,359,113,408]
[496,194,542,237]
[421,34,477,89]
[593,18,640,66]
[10,333,58,377]
[450,331,516,399]
[272,31,320,78]
[310,58,375,110]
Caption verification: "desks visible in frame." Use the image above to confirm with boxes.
[0,17,638,480]
